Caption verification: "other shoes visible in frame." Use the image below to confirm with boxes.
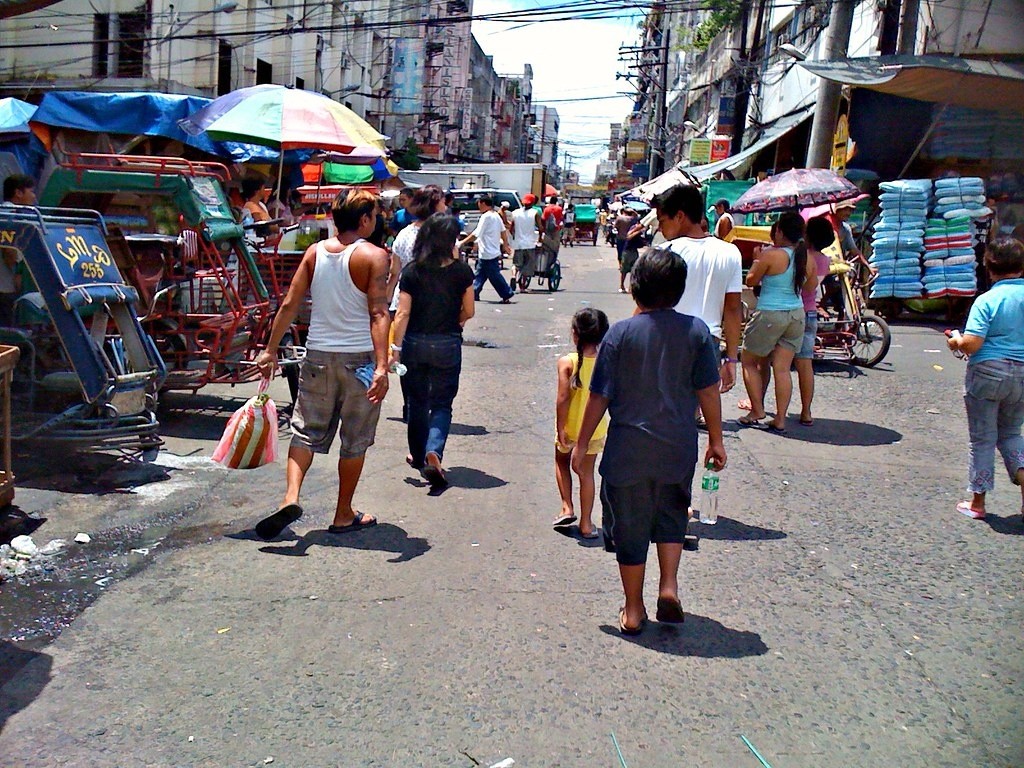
[499,294,515,304]
[618,288,627,293]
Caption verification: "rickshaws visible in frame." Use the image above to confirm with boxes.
[32,89,599,398]
[0,201,175,486]
[718,163,891,373]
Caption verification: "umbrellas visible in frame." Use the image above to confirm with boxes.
[178,83,401,233]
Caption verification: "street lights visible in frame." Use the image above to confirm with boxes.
[156,0,240,97]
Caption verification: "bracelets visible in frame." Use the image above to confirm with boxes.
[374,372,387,378]
[725,356,738,363]
[753,257,759,262]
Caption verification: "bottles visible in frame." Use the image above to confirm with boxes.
[389,361,407,376]
[296,214,329,248]
[699,463,719,525]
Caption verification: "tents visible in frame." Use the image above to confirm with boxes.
[795,51,1024,110]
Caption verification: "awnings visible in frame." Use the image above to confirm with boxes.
[630,106,816,204]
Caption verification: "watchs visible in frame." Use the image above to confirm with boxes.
[390,344,402,351]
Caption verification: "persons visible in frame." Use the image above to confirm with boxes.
[0,170,1023,635]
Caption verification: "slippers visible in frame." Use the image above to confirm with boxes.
[957,500,986,520]
[553,512,576,525]
[766,420,788,434]
[407,454,422,469]
[620,602,643,635]
[658,597,686,623]
[801,416,814,427]
[695,415,707,429]
[254,502,303,540]
[329,512,377,533]
[738,400,755,410]
[736,417,769,429]
[684,534,697,541]
[424,464,448,487]
[584,520,598,537]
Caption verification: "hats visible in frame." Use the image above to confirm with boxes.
[835,199,855,212]
[522,194,536,204]
[501,201,510,207]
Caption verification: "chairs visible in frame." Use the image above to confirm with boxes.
[0,224,249,410]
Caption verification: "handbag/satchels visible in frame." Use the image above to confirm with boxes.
[212,365,278,470]
[545,214,558,233]
